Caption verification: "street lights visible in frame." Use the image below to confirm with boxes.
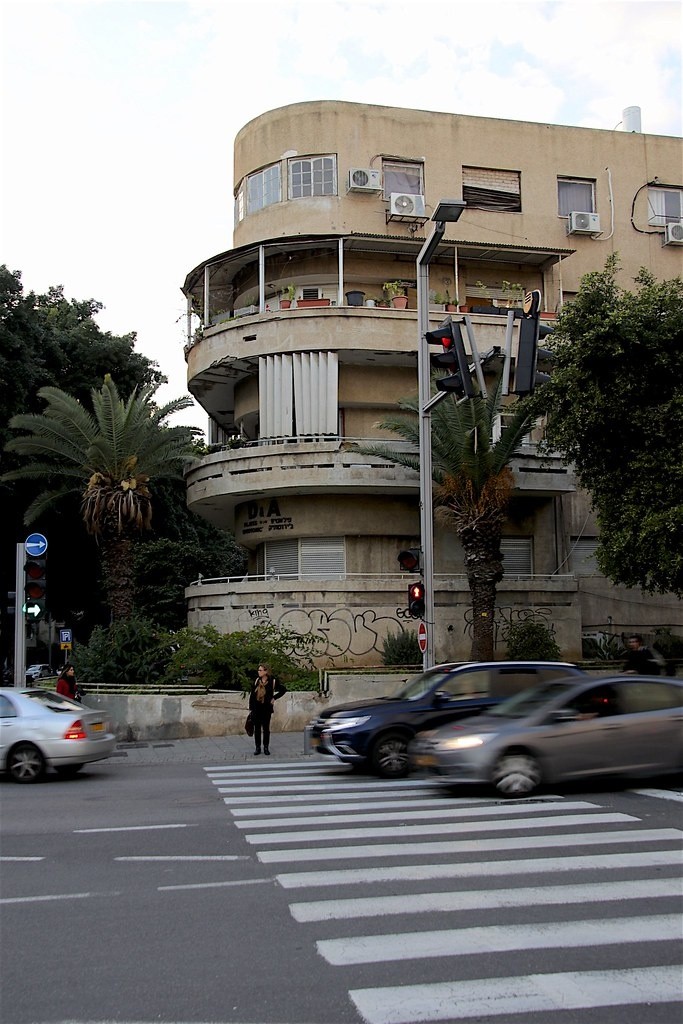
[414,197,472,676]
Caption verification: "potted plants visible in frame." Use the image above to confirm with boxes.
[470,281,525,316]
[374,281,408,309]
[211,309,232,323]
[280,281,297,308]
[429,290,458,312]
[234,296,257,319]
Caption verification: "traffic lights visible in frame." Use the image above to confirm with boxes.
[513,313,556,393]
[424,318,475,400]
[24,552,48,622]
[408,581,426,618]
[397,549,421,569]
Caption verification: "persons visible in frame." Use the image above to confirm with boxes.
[620,636,662,676]
[249,664,287,755]
[56,664,81,700]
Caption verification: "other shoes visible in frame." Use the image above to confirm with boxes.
[254,749,261,755]
[264,749,270,755]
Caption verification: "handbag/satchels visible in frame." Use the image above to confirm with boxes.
[75,695,81,703]
[245,710,255,736]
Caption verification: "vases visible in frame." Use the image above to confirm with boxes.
[297,299,330,307]
[540,311,556,317]
[345,291,366,305]
[459,306,468,312]
[365,299,374,307]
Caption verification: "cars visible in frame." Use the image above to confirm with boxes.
[0,664,64,686]
[404,674,682,797]
[0,686,116,782]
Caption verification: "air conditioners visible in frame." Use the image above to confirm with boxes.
[390,193,425,222]
[569,212,600,236]
[665,223,683,246]
[346,168,380,196]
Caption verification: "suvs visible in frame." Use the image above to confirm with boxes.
[307,659,622,779]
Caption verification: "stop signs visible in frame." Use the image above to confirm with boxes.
[418,622,427,653]
